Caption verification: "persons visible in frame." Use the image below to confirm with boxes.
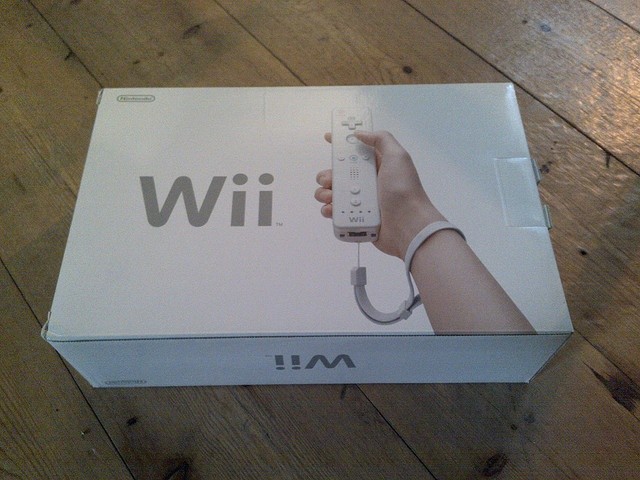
[314,131,536,335]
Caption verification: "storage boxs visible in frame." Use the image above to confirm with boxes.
[43,81,574,388]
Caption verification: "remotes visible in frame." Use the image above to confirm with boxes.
[330,104,383,244]
